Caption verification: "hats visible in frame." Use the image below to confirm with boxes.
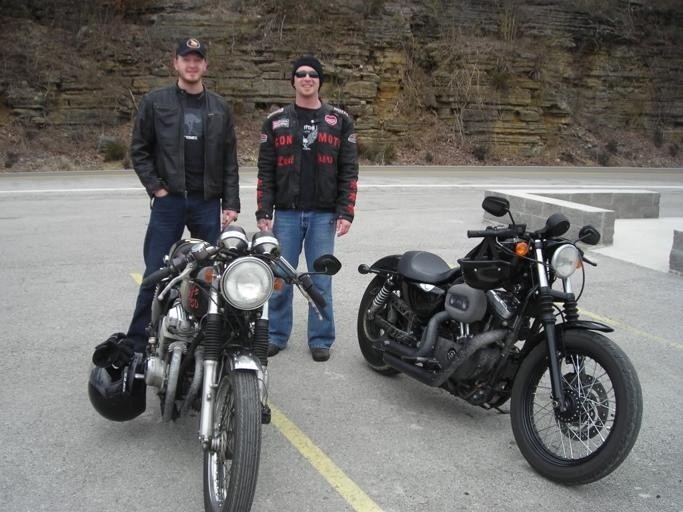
[290,56,325,91]
[177,38,208,59]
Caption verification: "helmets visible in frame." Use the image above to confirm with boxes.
[88,362,147,422]
[457,258,512,291]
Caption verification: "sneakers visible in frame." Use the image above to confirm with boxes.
[267,343,286,357]
[308,343,331,362]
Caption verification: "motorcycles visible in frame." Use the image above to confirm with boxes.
[355,194,643,488]
[134,225,341,512]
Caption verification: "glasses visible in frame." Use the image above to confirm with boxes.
[294,71,319,78]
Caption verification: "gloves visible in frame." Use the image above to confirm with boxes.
[92,333,136,369]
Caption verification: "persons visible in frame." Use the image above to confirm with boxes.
[125,36,240,357]
[255,54,360,362]
